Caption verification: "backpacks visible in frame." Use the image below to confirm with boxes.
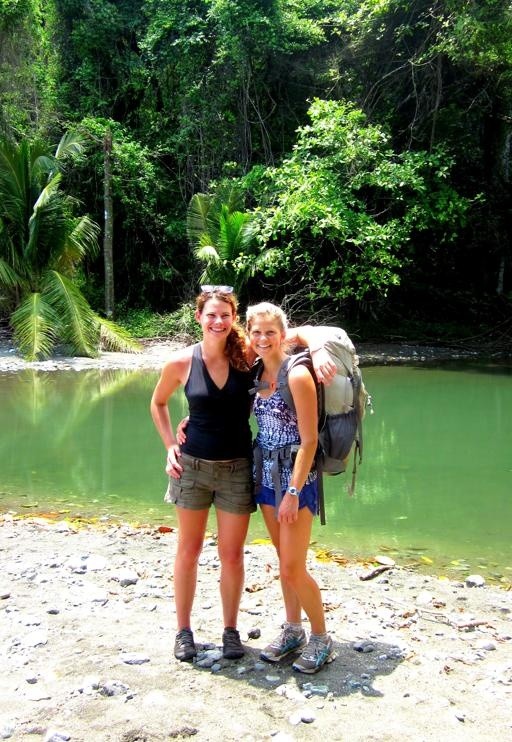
[276,327,368,476]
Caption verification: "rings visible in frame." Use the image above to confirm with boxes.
[177,433,179,436]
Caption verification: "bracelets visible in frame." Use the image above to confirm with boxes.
[310,344,324,356]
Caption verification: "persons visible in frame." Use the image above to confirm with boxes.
[150,285,337,662]
[176,300,337,674]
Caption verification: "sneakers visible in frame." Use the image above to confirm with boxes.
[292,634,336,674]
[260,623,306,660]
[174,630,197,660]
[222,627,244,658]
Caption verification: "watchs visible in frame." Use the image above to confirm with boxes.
[286,486,300,496]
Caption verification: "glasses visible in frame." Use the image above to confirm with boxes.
[200,285,234,294]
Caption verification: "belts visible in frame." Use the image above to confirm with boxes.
[181,456,250,474]
